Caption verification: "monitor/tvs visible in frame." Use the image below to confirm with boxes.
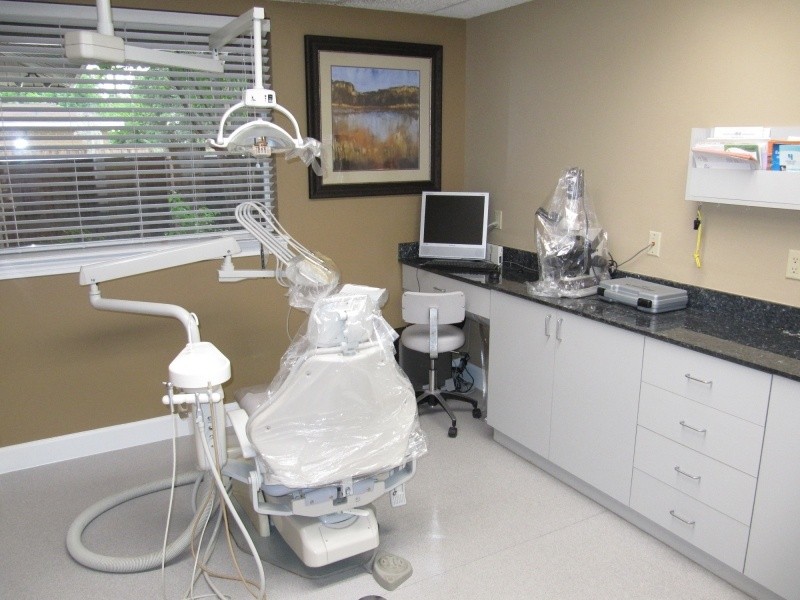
[418,191,489,261]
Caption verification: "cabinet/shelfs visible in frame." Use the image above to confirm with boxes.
[401,264,800,600]
[685,127,800,210]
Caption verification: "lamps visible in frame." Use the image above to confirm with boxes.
[64,0,303,158]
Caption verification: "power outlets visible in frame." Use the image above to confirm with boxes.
[647,232,661,256]
[786,250,800,279]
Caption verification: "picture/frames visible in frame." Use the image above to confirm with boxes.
[303,35,444,199]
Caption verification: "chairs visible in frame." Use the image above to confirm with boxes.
[400,291,482,437]
